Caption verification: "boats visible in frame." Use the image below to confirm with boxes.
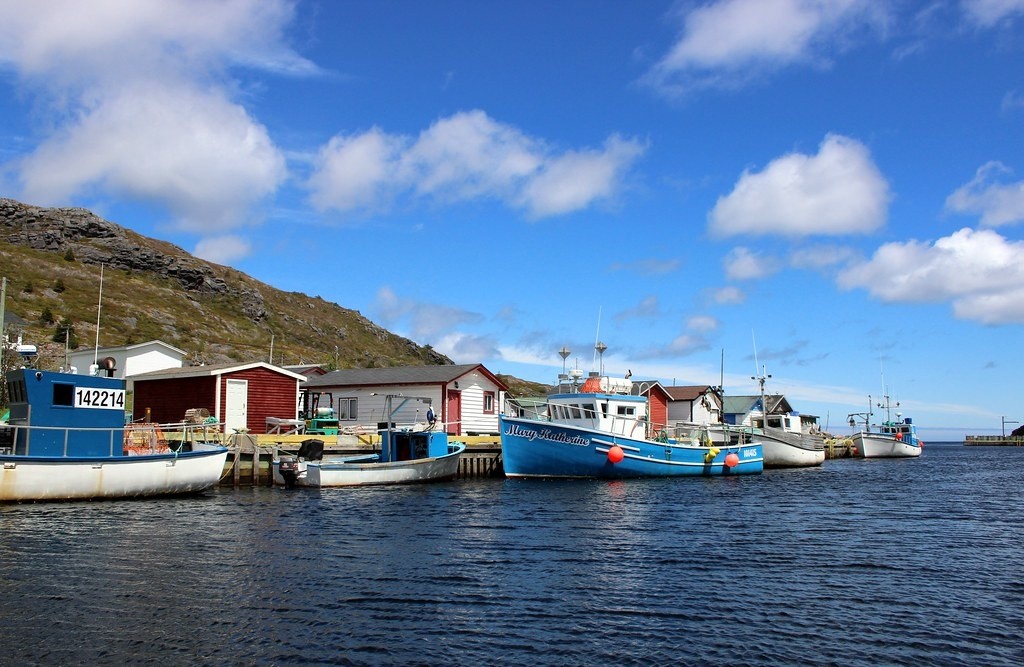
[500,304,764,479]
[272,397,469,489]
[850,353,924,458]
[0,259,229,505]
[747,326,826,467]
